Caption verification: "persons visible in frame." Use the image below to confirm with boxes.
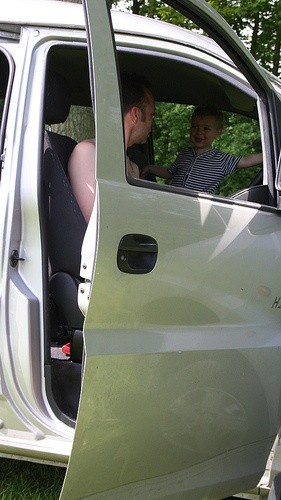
[66,69,156,223]
[141,103,264,195]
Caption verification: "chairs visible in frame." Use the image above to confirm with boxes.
[41,69,89,331]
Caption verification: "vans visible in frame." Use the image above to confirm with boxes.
[0,1,280,500]
